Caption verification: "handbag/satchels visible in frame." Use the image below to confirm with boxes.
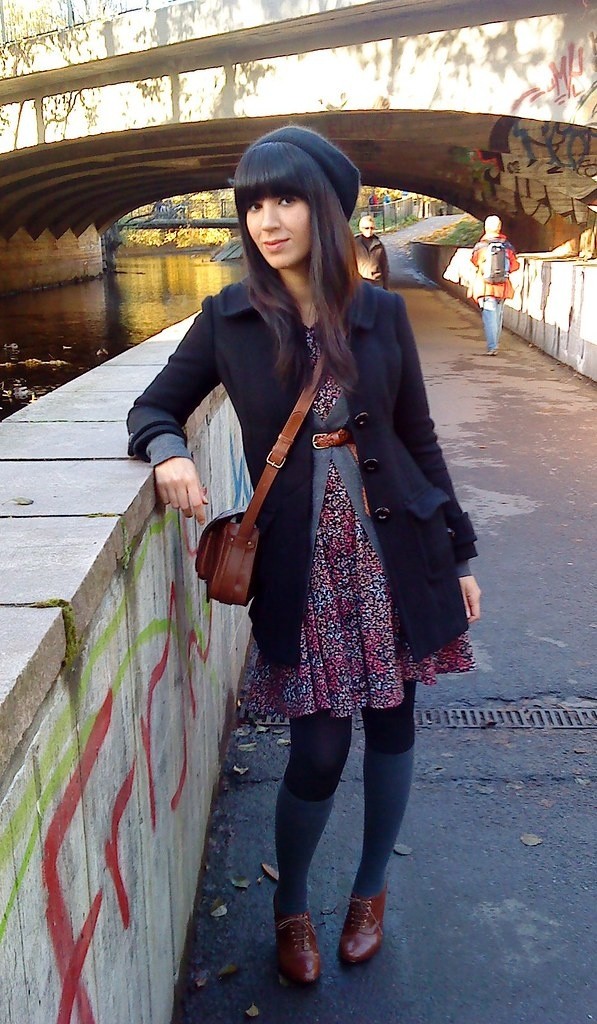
[194,507,262,608]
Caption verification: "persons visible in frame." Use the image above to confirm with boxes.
[382,191,390,204]
[369,190,378,217]
[126,126,480,984]
[471,215,520,355]
[152,199,172,213]
[354,215,389,290]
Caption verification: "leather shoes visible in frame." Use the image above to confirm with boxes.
[338,885,387,961]
[273,888,321,983]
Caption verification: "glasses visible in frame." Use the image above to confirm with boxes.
[362,227,374,230]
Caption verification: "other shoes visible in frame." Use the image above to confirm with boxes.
[486,348,500,356]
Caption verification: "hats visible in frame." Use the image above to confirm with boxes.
[250,126,360,222]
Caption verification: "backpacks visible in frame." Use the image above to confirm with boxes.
[484,241,509,284]
